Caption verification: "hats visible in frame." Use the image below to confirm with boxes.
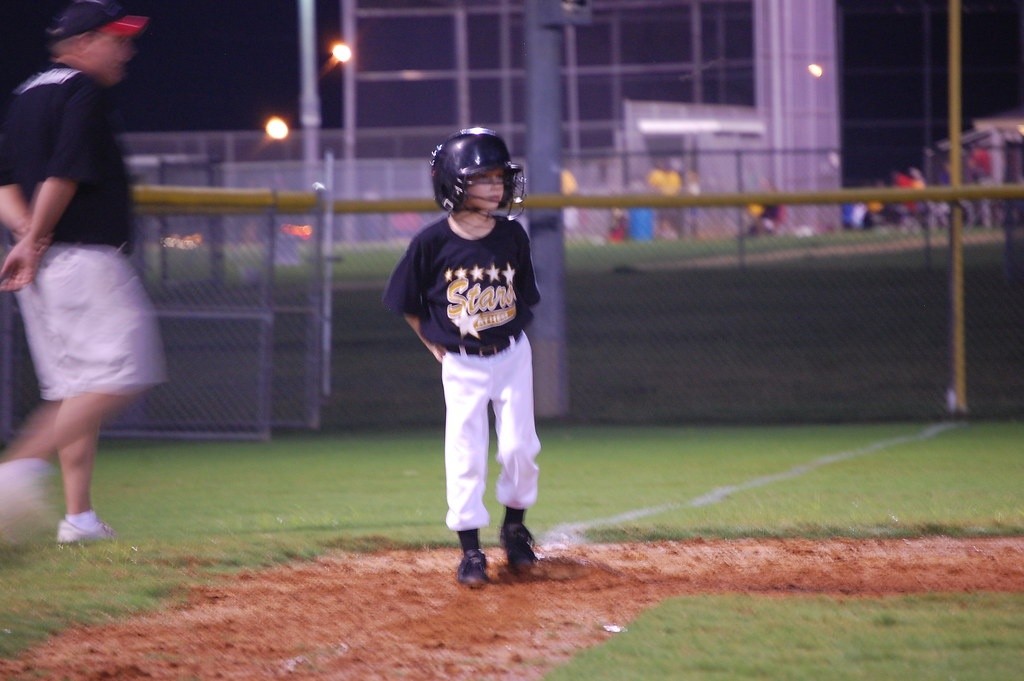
[48,0,149,43]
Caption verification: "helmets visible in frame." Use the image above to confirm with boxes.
[430,128,523,210]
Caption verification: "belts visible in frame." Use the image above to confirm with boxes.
[445,333,521,357]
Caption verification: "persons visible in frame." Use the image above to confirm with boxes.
[382,125,542,587]
[0,0,173,545]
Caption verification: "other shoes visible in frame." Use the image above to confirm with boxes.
[459,548,489,585]
[58,519,117,542]
[500,525,538,570]
